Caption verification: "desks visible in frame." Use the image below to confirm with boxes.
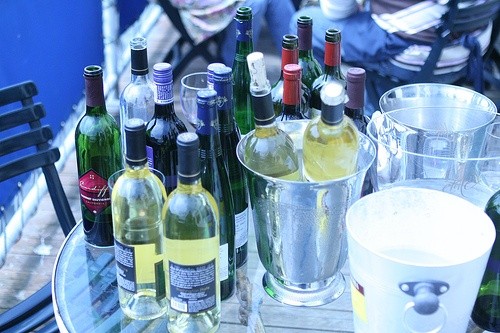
[50,195,500,333]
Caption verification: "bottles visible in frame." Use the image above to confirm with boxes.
[77,6,371,320]
[465,190,500,333]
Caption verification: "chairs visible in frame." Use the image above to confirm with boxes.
[0,78,170,333]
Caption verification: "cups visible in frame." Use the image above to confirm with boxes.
[346,82,500,333]
[108,166,167,198]
[180,72,209,128]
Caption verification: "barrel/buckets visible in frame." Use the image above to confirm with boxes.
[236,118,376,306]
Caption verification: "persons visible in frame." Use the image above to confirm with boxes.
[170,0,296,68]
[290,0,500,118]
